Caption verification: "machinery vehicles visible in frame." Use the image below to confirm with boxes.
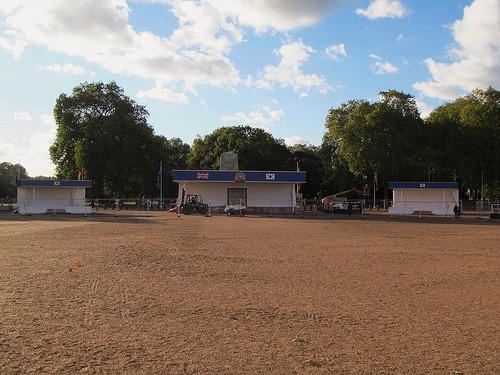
[224,198,247,216]
[317,187,362,214]
[182,193,209,215]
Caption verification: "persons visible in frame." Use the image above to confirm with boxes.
[347,203,353,216]
[303,198,323,212]
[358,199,364,216]
[188,196,196,205]
[453,205,458,218]
[90,195,169,212]
